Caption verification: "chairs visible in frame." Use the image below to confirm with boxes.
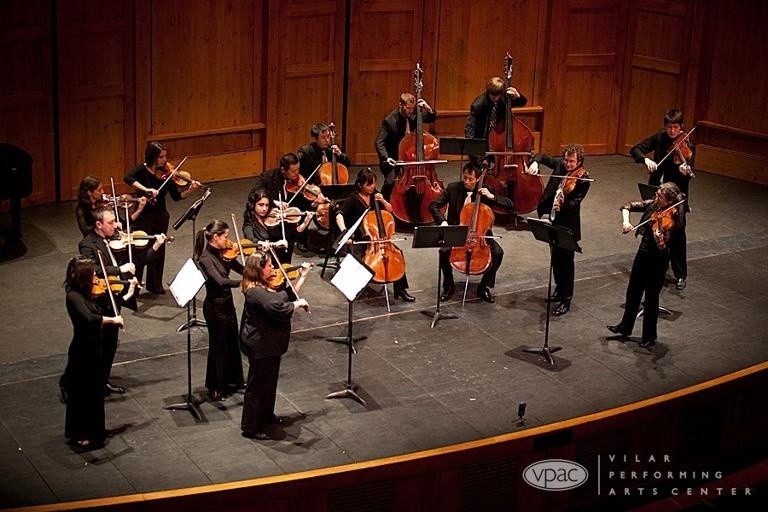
[319,198,347,277]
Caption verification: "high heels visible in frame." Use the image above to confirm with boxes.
[394,289,415,302]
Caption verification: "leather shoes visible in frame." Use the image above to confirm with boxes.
[607,324,632,335]
[677,277,686,290]
[244,414,283,439]
[105,383,125,393]
[639,339,654,347]
[545,295,569,316]
[477,287,494,303]
[441,286,455,302]
[209,382,247,401]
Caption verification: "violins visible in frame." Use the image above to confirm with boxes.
[264,256,313,291]
[551,120,696,252]
[91,269,142,297]
[265,206,317,225]
[221,236,286,262]
[97,157,206,254]
[286,170,332,207]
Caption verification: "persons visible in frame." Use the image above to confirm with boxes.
[465,77,528,164]
[239,251,308,439]
[335,167,416,302]
[607,182,688,348]
[375,93,437,201]
[243,122,350,274]
[527,143,590,317]
[194,219,246,400]
[58,176,149,452]
[123,141,200,295]
[630,107,695,290]
[429,164,515,303]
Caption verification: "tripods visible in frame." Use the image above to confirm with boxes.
[176,221,207,333]
[162,307,205,423]
[326,302,370,408]
[521,243,561,366]
[325,303,368,354]
[636,305,673,319]
[421,245,459,329]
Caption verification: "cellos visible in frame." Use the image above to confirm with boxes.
[362,168,409,283]
[391,81,449,225]
[490,83,545,213]
[451,161,493,277]
[319,120,347,184]
